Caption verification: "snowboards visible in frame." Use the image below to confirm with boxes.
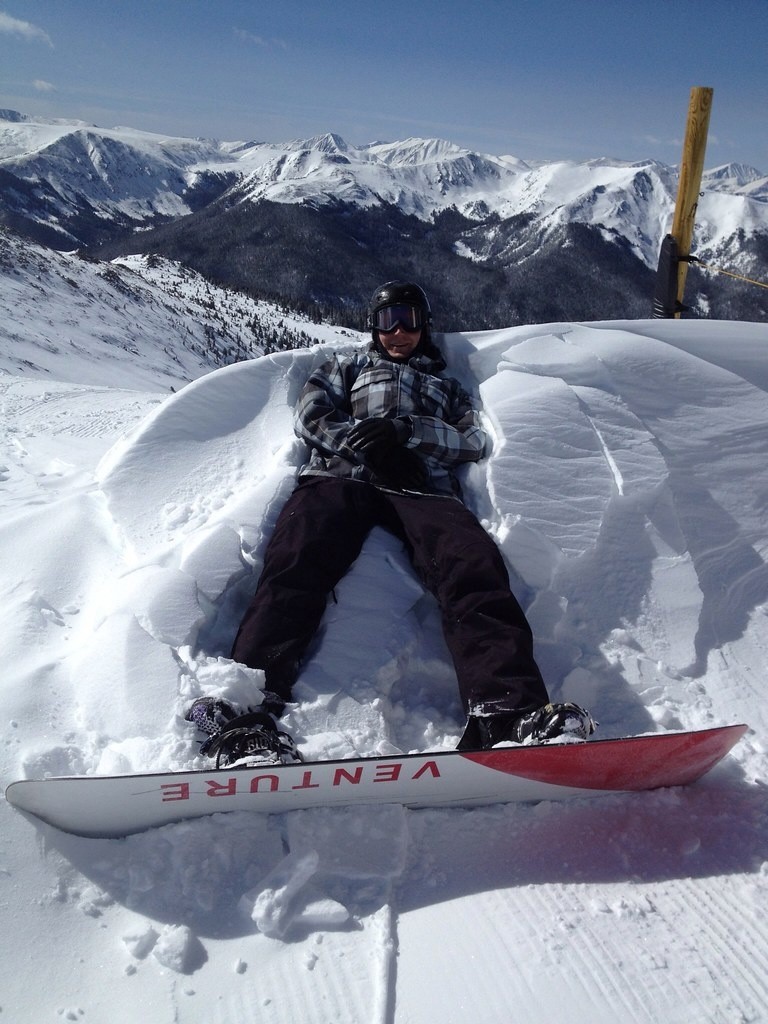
[4,722,749,838]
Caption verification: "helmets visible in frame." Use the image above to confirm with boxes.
[368,281,433,331]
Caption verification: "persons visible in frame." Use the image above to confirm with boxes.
[188,278,596,772]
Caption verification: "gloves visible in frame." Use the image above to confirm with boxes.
[348,416,408,450]
[365,447,431,497]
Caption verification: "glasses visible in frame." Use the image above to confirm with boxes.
[370,304,427,334]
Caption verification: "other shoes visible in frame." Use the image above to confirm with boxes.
[476,702,596,755]
[182,701,301,773]
